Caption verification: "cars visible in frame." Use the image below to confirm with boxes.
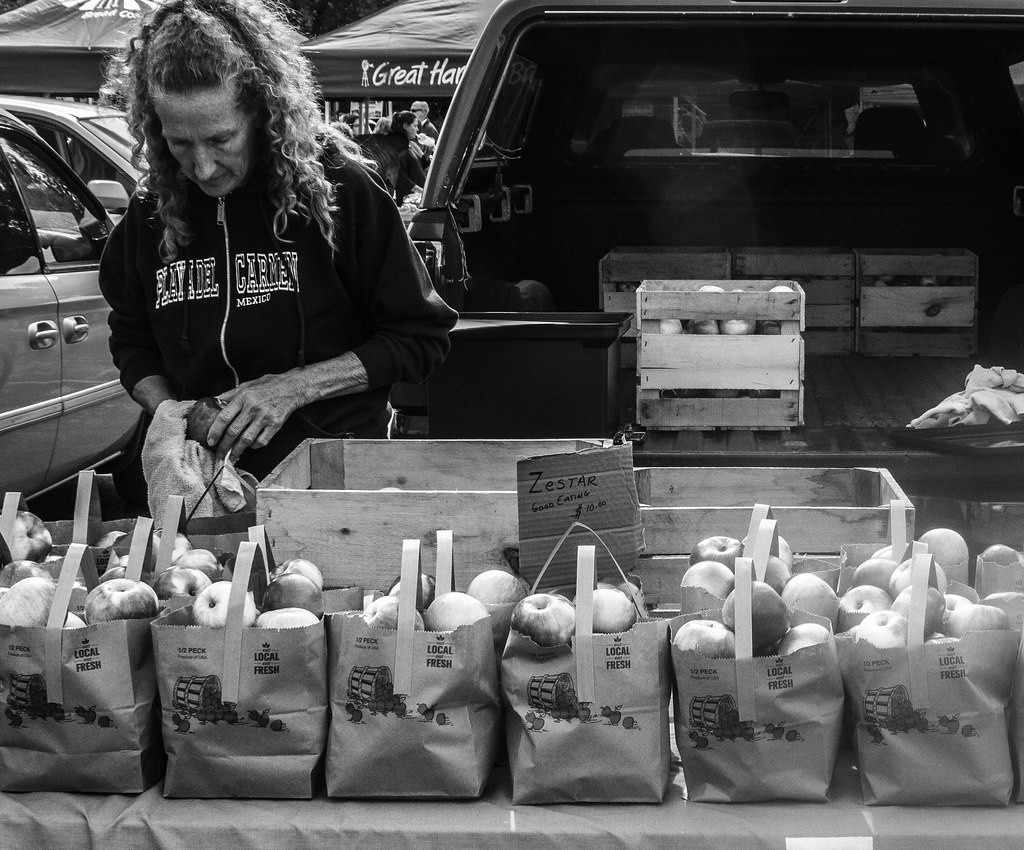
[0,94,152,518]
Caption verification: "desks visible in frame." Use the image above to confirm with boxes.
[1,723,1024,850]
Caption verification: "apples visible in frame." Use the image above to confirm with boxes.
[660,284,794,398]
[0,510,1024,663]
[186,397,229,450]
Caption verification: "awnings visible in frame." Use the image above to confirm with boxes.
[0,0,504,99]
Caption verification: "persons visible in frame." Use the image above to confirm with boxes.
[325,102,442,208]
[99,0,459,516]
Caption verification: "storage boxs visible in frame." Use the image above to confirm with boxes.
[253,437,616,593]
[634,277,808,432]
[597,247,730,340]
[633,463,916,612]
[857,244,987,360]
[734,248,857,355]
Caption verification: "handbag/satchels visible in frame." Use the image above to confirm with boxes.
[0,472,1024,805]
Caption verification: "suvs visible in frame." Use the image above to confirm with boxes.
[405,0,1023,500]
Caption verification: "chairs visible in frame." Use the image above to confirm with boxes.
[597,118,681,194]
[850,103,928,165]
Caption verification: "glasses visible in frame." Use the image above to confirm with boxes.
[411,109,425,112]
